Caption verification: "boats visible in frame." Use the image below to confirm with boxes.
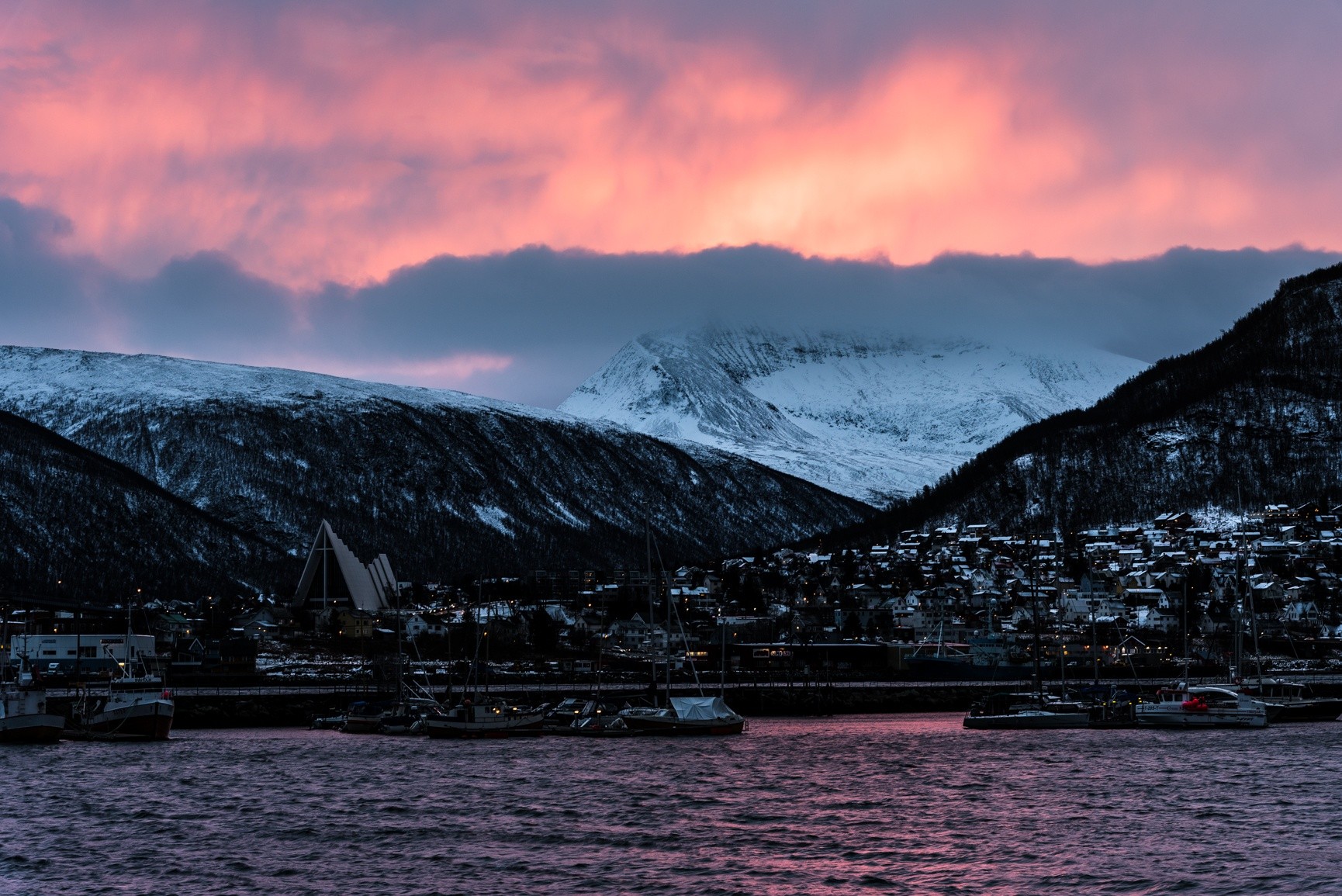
[0,616,68,745]
[69,589,176,742]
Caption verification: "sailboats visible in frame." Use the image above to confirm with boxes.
[960,478,1342,730]
[308,504,746,740]
